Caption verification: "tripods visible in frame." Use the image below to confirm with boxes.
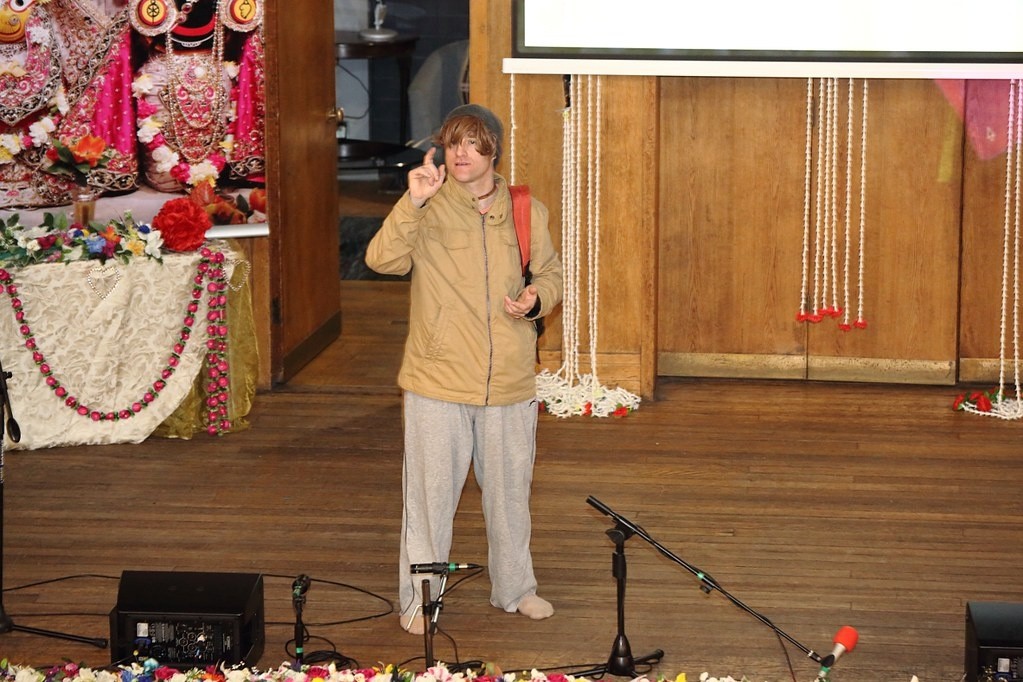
[562,494,822,680]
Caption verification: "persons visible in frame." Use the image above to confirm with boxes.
[364,104,562,636]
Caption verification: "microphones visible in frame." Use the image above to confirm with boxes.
[410,561,480,574]
[291,574,311,598]
[814,626,858,682]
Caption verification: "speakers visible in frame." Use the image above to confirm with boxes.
[963,600,1023,682]
[108,570,266,674]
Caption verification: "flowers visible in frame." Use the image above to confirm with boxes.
[0,649,743,682]
[0,209,165,276]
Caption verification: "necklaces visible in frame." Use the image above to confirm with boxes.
[478,184,497,200]
